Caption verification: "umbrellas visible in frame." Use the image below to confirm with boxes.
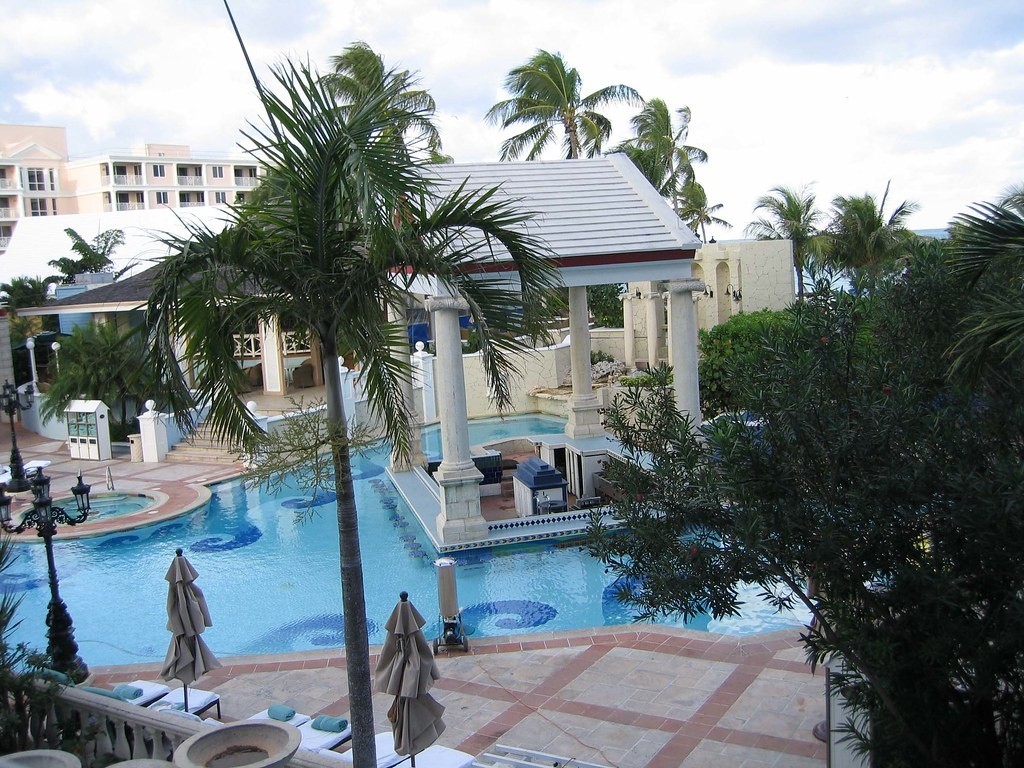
[155,548,223,713]
[374,591,447,767]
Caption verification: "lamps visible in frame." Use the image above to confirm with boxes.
[702,285,713,298]
[733,288,742,305]
[724,283,733,298]
[636,287,641,299]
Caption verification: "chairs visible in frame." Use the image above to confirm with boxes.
[319,732,410,768]
[81,679,171,708]
[0,460,51,483]
[297,719,352,753]
[146,686,222,719]
[392,745,478,768]
[204,709,311,727]
